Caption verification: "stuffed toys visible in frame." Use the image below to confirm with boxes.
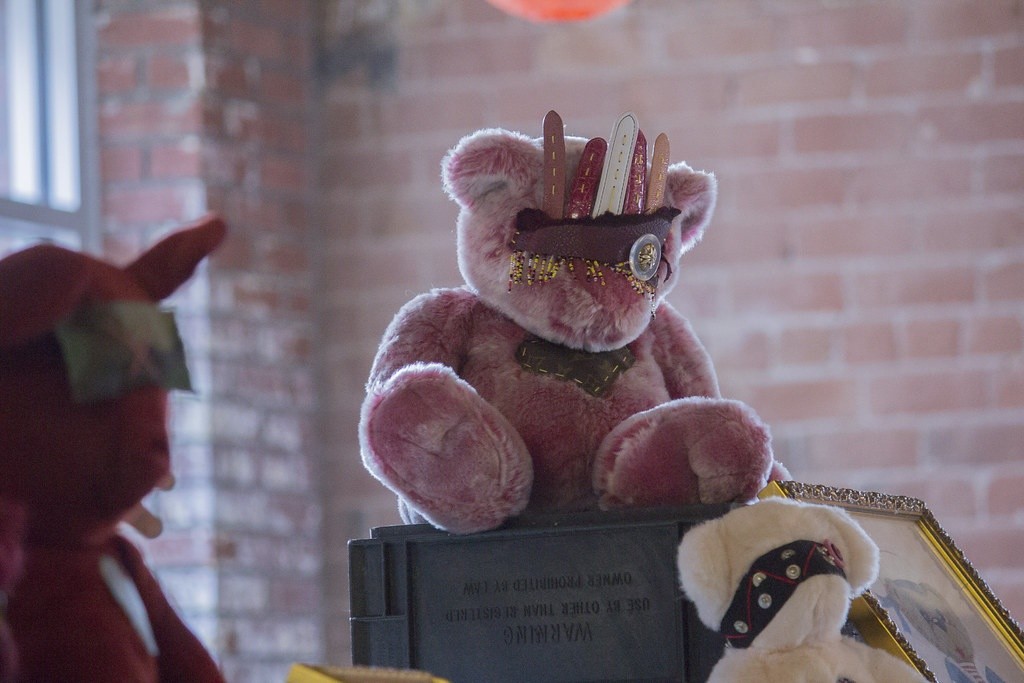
[675,497,930,682]
[0,217,234,683]
[358,111,788,540]
